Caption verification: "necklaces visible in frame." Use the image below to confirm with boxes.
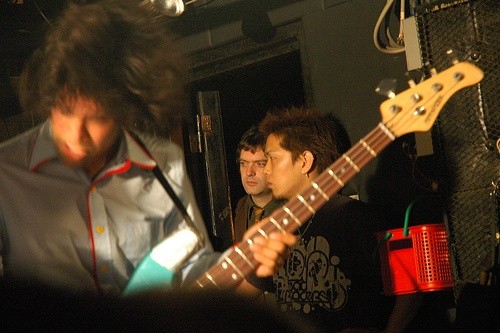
[292,209,316,248]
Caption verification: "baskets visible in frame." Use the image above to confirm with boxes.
[372,194,455,296]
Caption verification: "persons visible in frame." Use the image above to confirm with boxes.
[236,104,421,333]
[219,125,287,259]
[0,0,300,299]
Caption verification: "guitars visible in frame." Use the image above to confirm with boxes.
[122,60,483,297]
